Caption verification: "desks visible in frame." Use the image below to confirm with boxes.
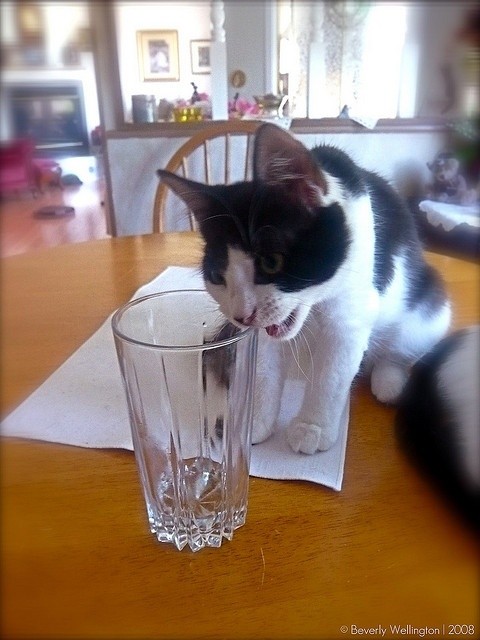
[0,231,479,640]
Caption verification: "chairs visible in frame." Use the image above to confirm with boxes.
[153,120,257,233]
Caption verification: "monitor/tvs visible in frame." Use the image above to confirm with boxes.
[0,81,91,158]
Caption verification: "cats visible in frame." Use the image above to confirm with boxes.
[155,122,453,455]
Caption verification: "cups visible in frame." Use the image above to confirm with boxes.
[111,288,258,548]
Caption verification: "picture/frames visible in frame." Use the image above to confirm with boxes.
[138,29,180,82]
[189,38,213,74]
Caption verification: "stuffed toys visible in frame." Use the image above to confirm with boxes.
[426,158,476,208]
